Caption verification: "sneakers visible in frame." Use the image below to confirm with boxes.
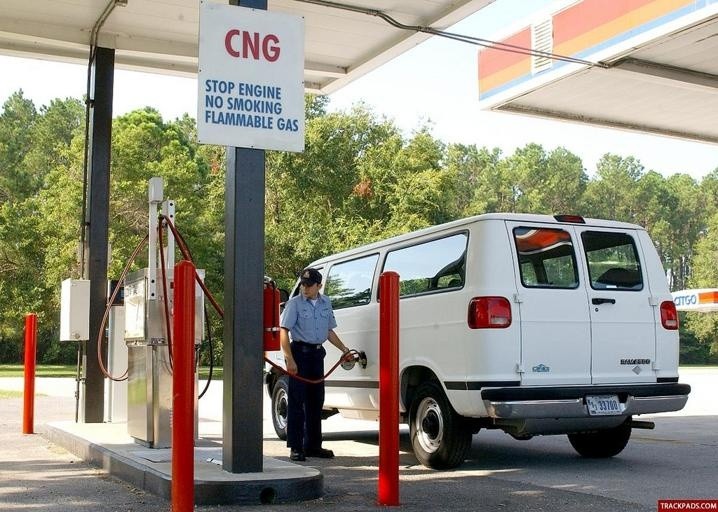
[304,448,335,459]
[290,448,306,462]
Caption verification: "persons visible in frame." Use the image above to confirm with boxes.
[279,266,353,462]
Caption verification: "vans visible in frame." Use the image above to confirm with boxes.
[266,212,693,471]
[670,287,718,312]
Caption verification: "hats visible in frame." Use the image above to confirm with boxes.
[299,268,323,285]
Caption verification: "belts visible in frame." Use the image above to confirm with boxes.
[293,340,323,349]
